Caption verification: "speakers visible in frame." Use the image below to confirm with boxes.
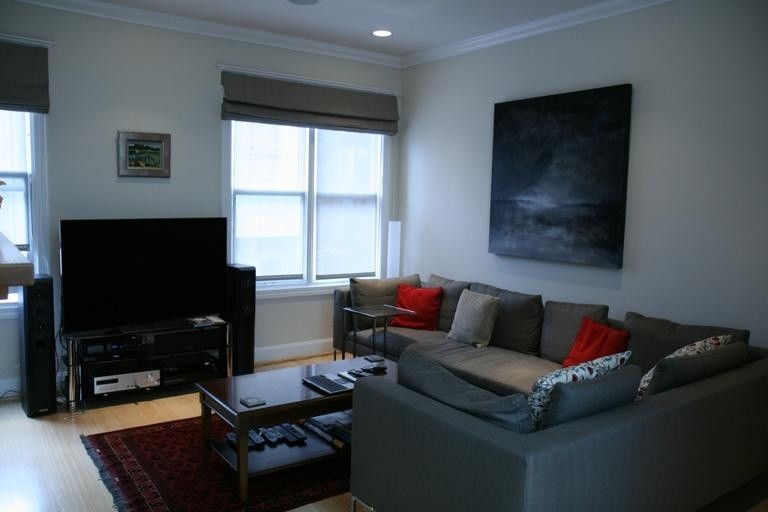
[226,264,255,376]
[18,273,57,418]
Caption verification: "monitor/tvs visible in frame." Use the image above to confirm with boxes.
[60,217,227,334]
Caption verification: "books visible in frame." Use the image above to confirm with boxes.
[305,408,352,445]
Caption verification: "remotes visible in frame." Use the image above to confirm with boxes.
[348,369,376,377]
[248,423,308,447]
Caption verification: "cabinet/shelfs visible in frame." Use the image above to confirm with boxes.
[59,316,234,414]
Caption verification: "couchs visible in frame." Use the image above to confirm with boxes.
[333,282,767,512]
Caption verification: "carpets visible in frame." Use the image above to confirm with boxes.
[79,414,351,512]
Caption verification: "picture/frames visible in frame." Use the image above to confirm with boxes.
[116,131,172,179]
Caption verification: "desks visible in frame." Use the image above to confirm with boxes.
[341,303,417,361]
